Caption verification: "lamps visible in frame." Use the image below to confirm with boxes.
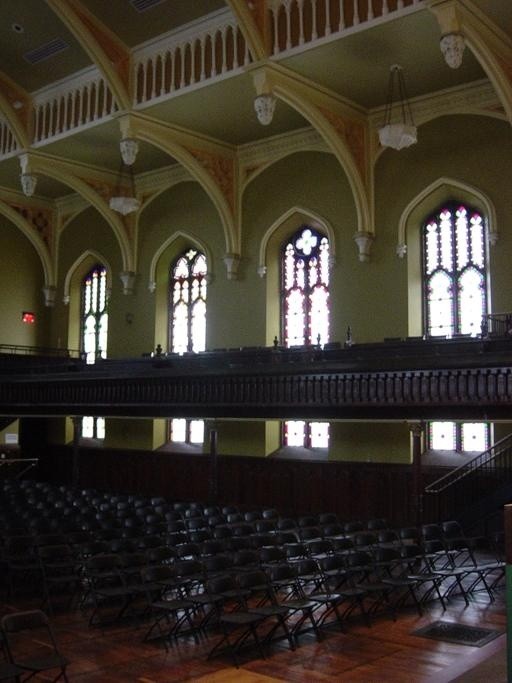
[20,174,38,198]
[118,138,140,166]
[439,30,466,70]
[109,153,140,216]
[253,93,277,126]
[378,65,420,152]
